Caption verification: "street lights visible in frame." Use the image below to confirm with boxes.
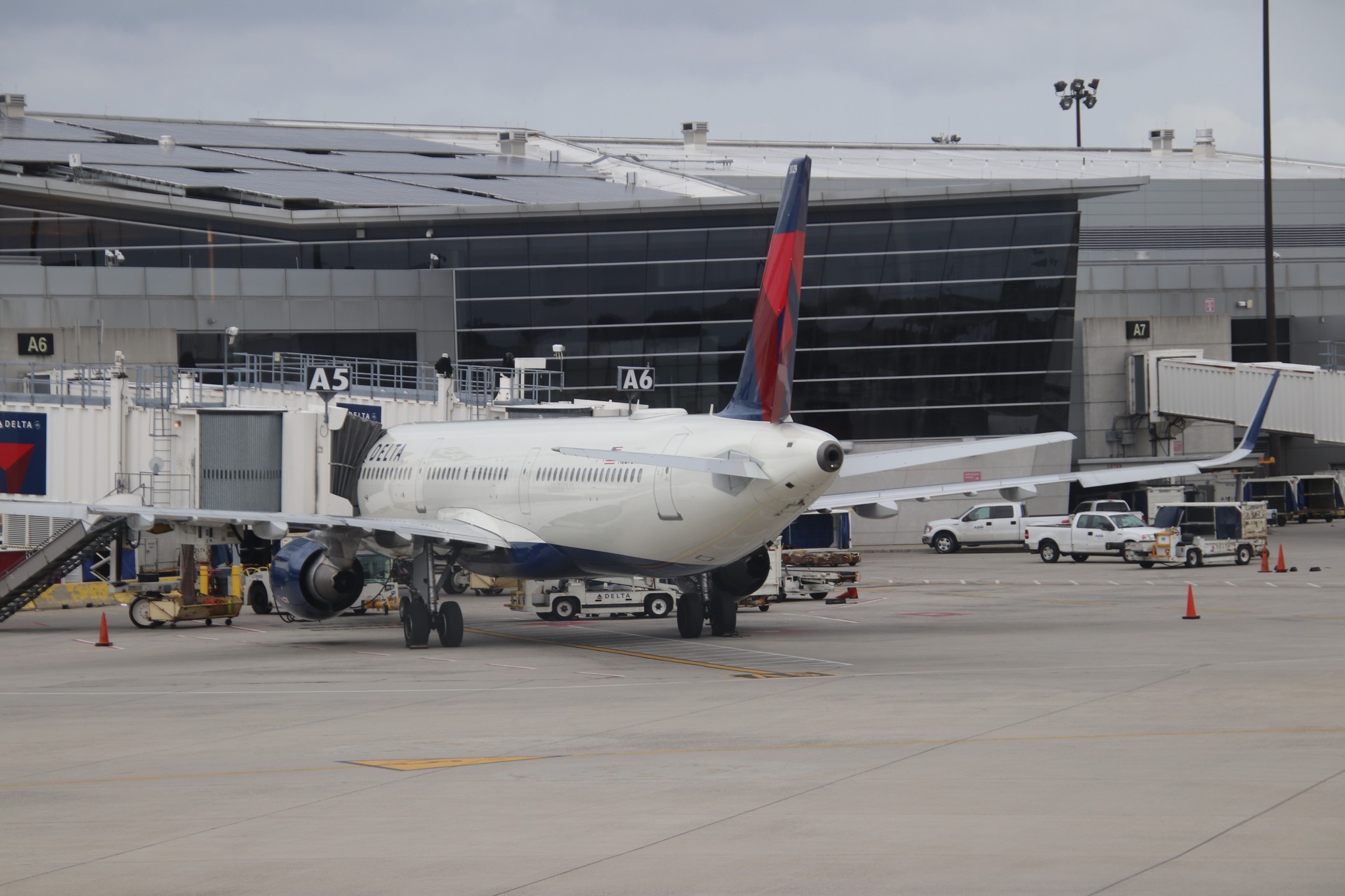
[223,326,238,407]
[1053,77,1100,149]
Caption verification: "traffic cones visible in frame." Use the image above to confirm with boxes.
[1181,584,1201,619]
[1276,543,1287,572]
[1258,544,1273,572]
[94,612,113,647]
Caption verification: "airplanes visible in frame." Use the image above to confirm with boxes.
[85,156,1283,650]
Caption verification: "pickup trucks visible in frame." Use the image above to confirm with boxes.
[922,501,1076,554]
[1072,500,1145,528]
[1024,511,1163,563]
